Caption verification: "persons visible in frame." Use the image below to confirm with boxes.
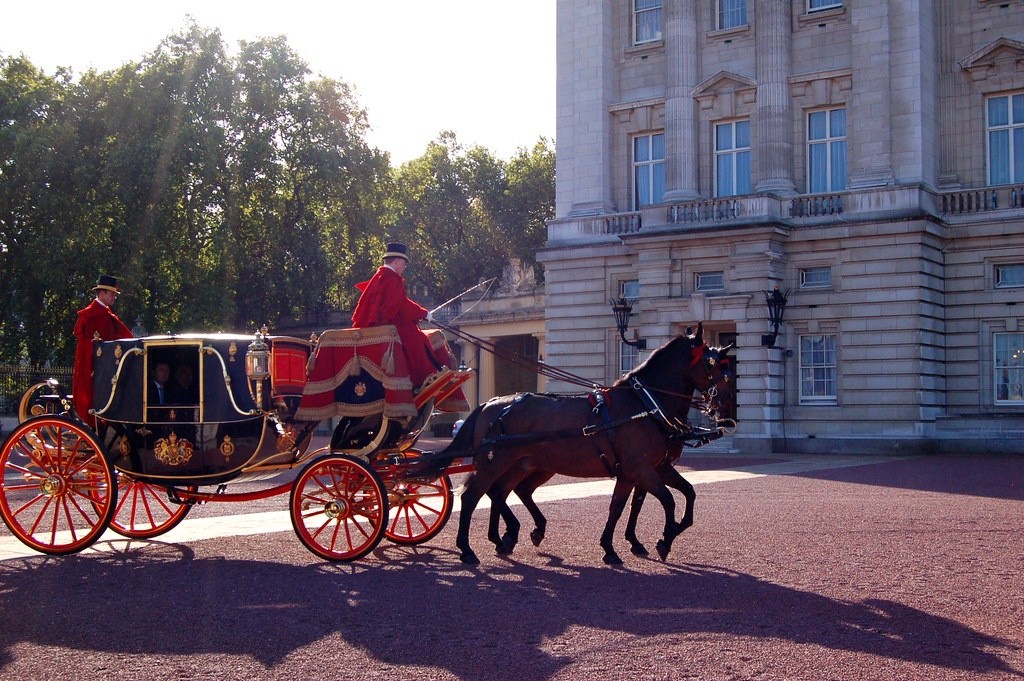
[352,243,459,388]
[74,273,135,428]
[148,360,195,405]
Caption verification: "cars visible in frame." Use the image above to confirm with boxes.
[452,419,465,440]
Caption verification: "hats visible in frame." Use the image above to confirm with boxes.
[92,275,121,294]
[382,243,410,263]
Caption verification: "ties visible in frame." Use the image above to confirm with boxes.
[159,388,164,405]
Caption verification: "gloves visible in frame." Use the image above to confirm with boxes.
[425,311,433,322]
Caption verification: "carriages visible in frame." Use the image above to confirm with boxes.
[0,318,741,565]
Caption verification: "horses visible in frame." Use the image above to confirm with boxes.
[407,320,735,566]
